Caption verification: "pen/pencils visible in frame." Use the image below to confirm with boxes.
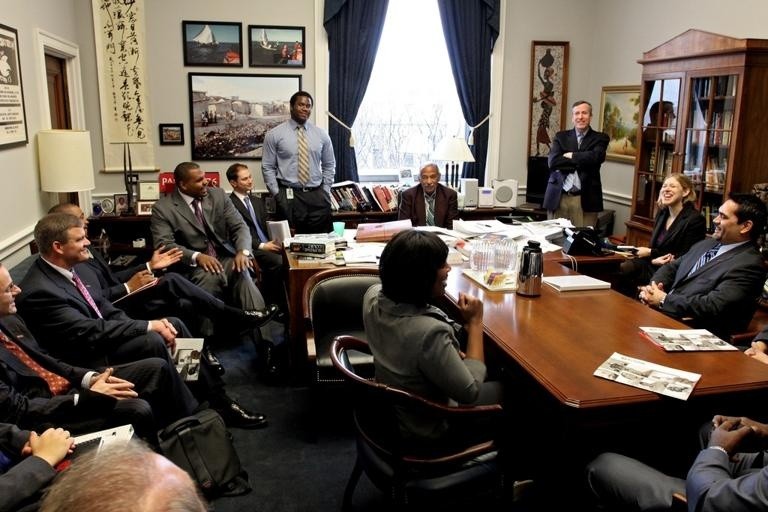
[477,223,492,228]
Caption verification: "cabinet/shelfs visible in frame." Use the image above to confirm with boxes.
[624,61,768,232]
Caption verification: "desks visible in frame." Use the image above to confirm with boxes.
[90,204,155,258]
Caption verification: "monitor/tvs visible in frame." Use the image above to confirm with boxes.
[526,156,555,212]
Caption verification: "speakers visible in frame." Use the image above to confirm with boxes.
[491,179,519,208]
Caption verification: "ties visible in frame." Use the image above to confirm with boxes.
[73,272,104,319]
[191,199,217,258]
[0,330,71,396]
[244,197,268,243]
[562,132,585,192]
[688,243,722,276]
[426,198,435,226]
[297,125,309,186]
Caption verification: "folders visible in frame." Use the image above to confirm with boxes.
[543,274,612,292]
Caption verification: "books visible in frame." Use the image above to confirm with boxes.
[647,75,738,192]
[328,181,398,212]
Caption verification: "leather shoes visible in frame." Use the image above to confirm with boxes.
[216,402,274,429]
[236,303,278,335]
[206,350,225,373]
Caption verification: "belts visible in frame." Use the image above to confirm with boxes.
[562,190,581,196]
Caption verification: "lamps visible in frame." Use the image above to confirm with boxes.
[36,129,97,194]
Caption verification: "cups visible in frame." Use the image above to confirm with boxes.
[332,222,345,238]
[469,237,516,274]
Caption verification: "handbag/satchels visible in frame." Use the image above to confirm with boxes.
[157,409,240,492]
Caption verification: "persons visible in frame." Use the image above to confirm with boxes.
[637,100,677,171]
[259,88,339,232]
[397,163,461,230]
[582,410,766,512]
[360,226,509,453]
[614,169,709,297]
[1,162,295,511]
[742,326,768,365]
[535,97,612,230]
[200,110,230,128]
[634,191,768,343]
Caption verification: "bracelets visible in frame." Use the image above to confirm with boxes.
[658,294,668,311]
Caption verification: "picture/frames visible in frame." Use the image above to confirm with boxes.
[530,39,571,158]
[596,79,643,165]
[158,20,309,164]
[0,23,29,150]
[113,194,156,215]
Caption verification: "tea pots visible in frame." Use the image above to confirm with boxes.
[516,240,543,298]
